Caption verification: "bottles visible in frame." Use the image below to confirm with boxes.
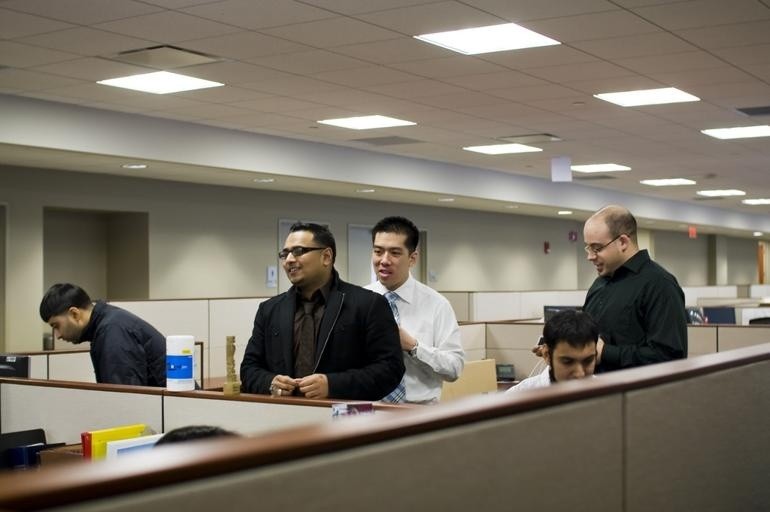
[166,335,195,392]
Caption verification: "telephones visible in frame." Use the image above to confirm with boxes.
[496,364,515,381]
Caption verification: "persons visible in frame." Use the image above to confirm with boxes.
[506,309,598,392]
[362,216,466,405]
[239,221,406,401]
[582,203,687,373]
[39,283,200,390]
[153,425,237,445]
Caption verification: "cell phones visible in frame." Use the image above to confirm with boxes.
[536,335,549,350]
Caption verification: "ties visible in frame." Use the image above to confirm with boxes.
[294,302,315,378]
[377,292,406,404]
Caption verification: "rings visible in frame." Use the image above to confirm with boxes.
[277,389,282,396]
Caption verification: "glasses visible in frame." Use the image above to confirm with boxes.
[278,246,325,260]
[584,233,630,253]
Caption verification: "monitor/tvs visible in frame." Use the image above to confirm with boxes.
[703,305,734,324]
[543,305,587,321]
[106,434,162,459]
[0,353,30,377]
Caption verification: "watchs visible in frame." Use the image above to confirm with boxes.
[408,339,419,357]
